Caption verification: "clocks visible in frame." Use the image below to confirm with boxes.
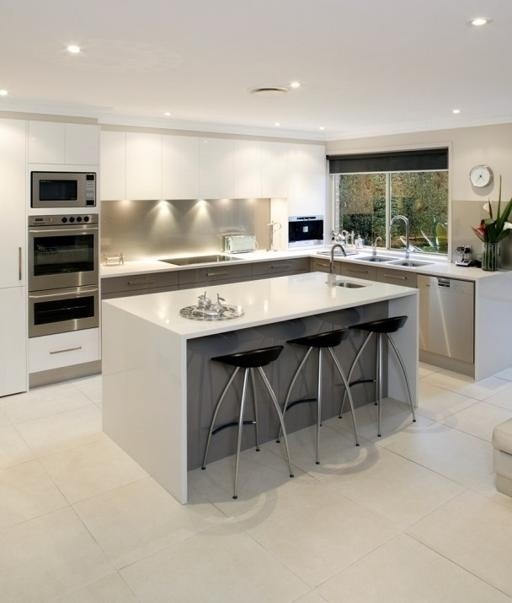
[469,165,494,187]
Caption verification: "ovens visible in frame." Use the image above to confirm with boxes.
[26,214,98,338]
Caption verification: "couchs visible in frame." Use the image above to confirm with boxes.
[492,419,512,497]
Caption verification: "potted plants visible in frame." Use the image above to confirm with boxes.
[471,174,512,271]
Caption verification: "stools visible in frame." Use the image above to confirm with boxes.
[276,327,360,465]
[339,314,417,438]
[202,345,294,500]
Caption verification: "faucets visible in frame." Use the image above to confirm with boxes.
[389,214,411,260]
[328,242,346,286]
[371,236,382,255]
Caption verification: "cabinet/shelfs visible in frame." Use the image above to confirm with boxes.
[252,254,310,280]
[26,115,97,173]
[0,111,28,398]
[416,264,512,381]
[340,259,376,281]
[27,327,105,390]
[101,270,179,300]
[178,262,252,289]
[310,255,341,274]
[376,263,416,288]
[99,124,289,198]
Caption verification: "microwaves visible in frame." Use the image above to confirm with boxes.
[30,171,97,208]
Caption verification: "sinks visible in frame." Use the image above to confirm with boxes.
[388,260,435,267]
[354,256,398,262]
[158,253,245,266]
[325,281,366,289]
